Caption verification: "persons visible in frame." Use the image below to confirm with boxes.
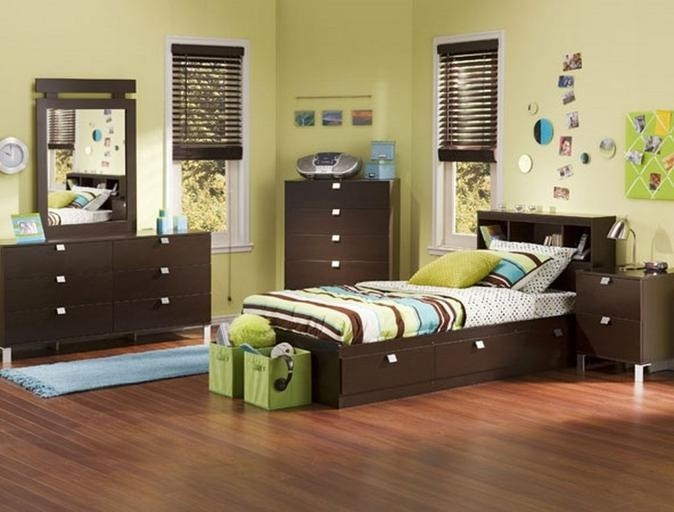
[559,137,572,157]
[564,53,581,70]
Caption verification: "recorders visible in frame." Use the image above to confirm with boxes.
[297,153,362,180]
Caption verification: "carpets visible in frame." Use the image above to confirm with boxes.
[0,343,210,398]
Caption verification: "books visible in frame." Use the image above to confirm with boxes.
[478,222,507,250]
[543,233,561,247]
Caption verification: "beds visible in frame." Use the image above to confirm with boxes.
[47,208,112,226]
[240,281,576,409]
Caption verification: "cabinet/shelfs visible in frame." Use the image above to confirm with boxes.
[112,231,212,344]
[0,239,113,363]
[284,180,400,290]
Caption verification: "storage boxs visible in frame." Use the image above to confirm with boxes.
[363,140,396,180]
[243,347,312,411]
[209,341,244,398]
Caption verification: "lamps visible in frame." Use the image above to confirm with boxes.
[607,218,646,271]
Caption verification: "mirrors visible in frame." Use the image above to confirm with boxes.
[35,78,137,240]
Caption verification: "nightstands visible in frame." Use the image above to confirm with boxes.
[474,211,615,293]
[66,173,126,208]
[576,264,674,382]
[112,198,126,220]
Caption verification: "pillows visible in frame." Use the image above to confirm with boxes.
[475,248,551,289]
[47,191,77,208]
[489,238,577,294]
[71,186,111,209]
[409,251,500,288]
[68,189,102,208]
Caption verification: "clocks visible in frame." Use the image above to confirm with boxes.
[0,137,29,174]
